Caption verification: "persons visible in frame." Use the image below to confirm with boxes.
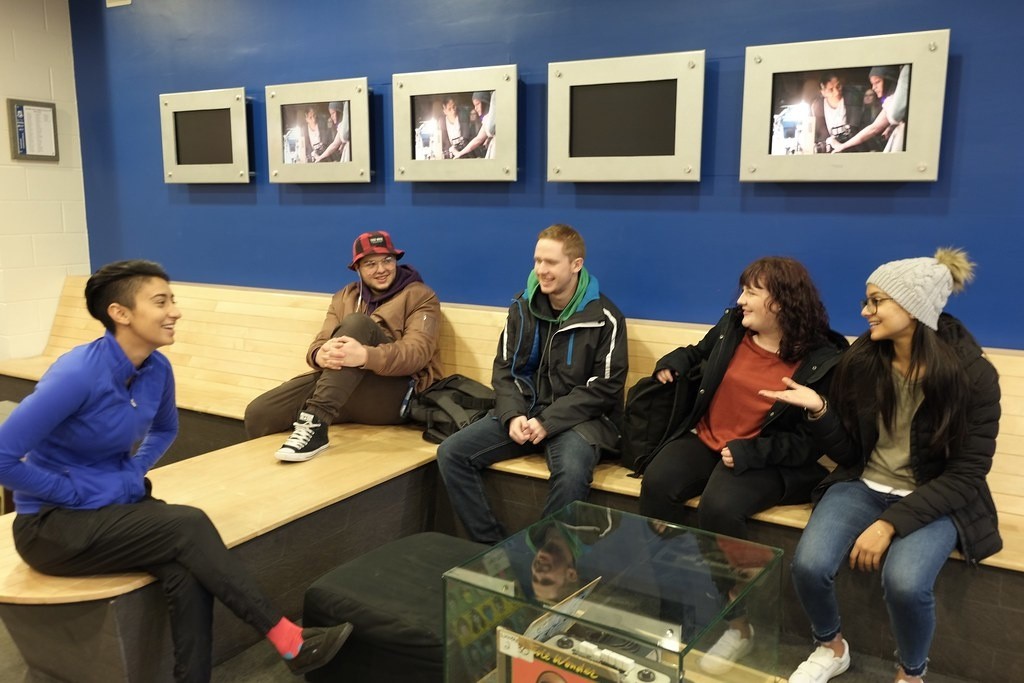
[440,90,496,159]
[639,258,848,676]
[437,225,628,546]
[245,231,443,463]
[526,500,623,602]
[1,260,354,682]
[304,101,350,162]
[758,248,1003,683]
[814,64,911,153]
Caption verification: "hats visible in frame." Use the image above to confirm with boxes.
[348,231,407,272]
[865,246,977,331]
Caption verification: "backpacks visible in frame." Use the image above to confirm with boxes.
[618,368,705,478]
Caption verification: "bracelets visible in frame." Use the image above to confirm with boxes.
[815,397,825,413]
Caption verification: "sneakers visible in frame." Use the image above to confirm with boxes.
[276,410,330,461]
[701,624,756,674]
[284,621,354,676]
[789,639,851,683]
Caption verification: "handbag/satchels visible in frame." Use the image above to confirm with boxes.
[409,374,498,445]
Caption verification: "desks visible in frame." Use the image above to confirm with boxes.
[443,502,784,683]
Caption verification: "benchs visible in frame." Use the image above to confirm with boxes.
[0,252,1023,683]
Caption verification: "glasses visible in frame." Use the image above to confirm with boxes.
[358,255,396,270]
[860,297,893,316]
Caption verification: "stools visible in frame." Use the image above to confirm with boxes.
[300,530,497,682]
[1,400,23,510]
